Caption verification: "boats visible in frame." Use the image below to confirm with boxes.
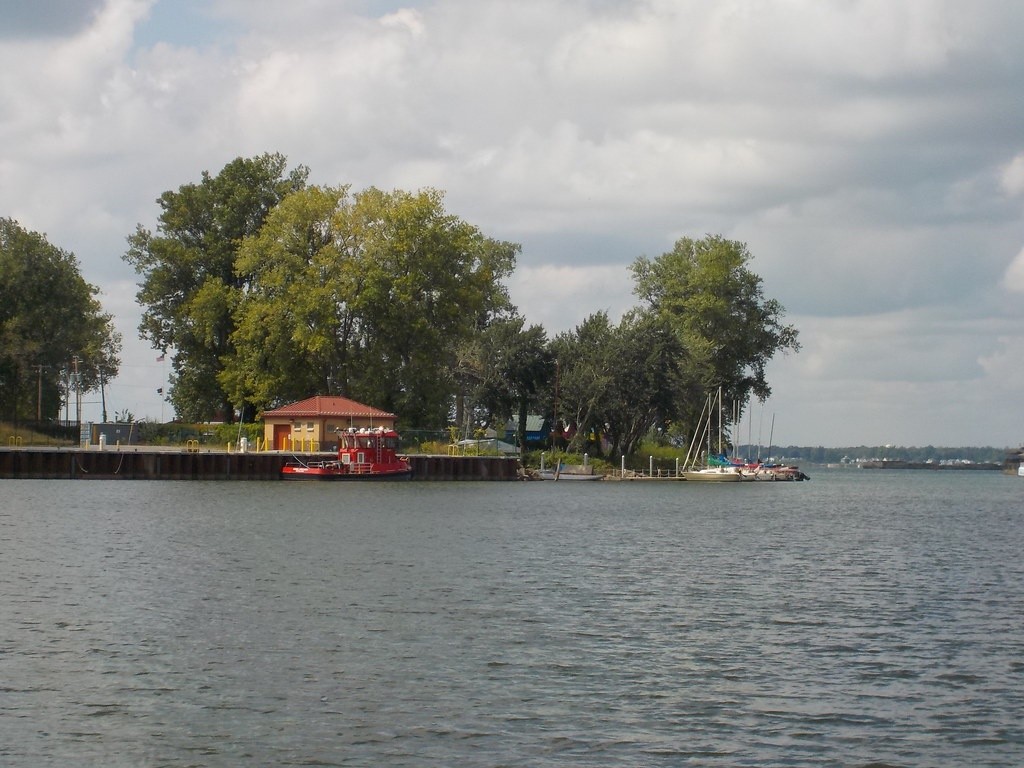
[279,426,413,481]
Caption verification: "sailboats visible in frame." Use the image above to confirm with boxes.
[680,385,810,482]
